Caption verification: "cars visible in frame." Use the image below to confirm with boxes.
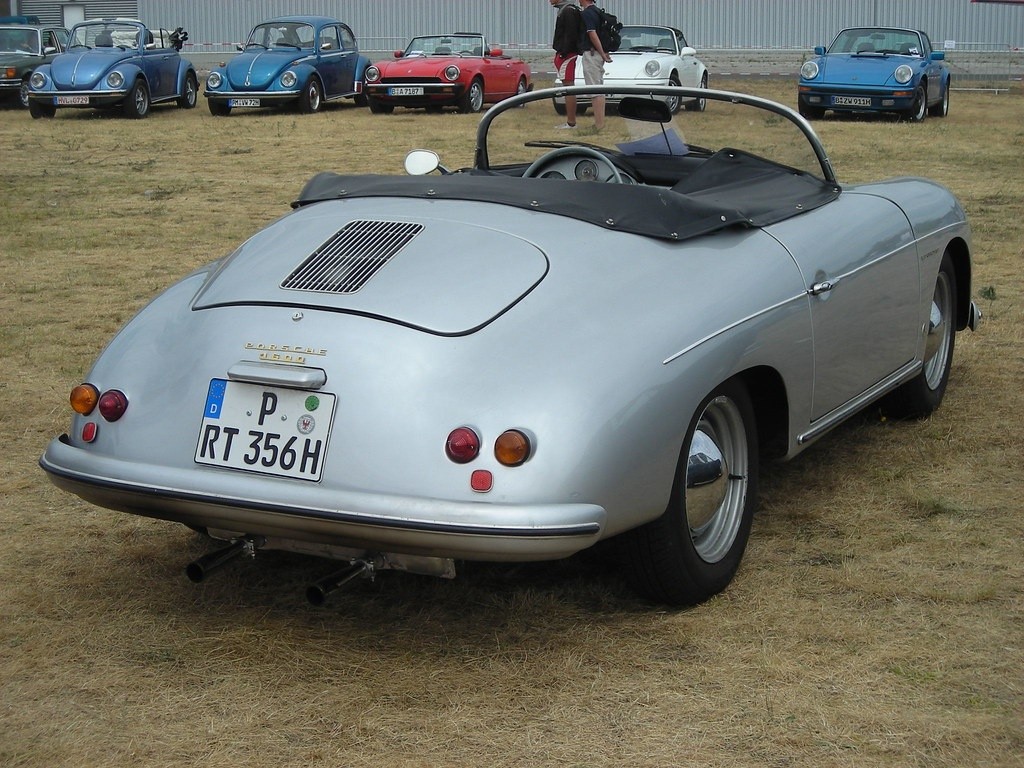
[203,15,372,116]
[25,18,199,119]
[0,24,83,110]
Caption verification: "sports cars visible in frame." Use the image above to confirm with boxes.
[364,31,533,114]
[551,24,709,113]
[37,84,982,606]
[797,26,952,123]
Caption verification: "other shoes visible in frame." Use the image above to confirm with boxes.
[554,122,577,130]
[575,123,609,136]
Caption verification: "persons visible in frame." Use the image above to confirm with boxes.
[549,0,613,133]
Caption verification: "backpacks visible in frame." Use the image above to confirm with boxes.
[557,4,587,55]
[589,5,623,53]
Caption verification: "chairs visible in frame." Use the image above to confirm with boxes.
[658,39,675,48]
[137,29,154,44]
[434,47,452,52]
[277,38,292,43]
[474,47,491,55]
[94,34,113,47]
[620,39,632,47]
[900,43,918,52]
[858,43,874,51]
[319,37,334,44]
